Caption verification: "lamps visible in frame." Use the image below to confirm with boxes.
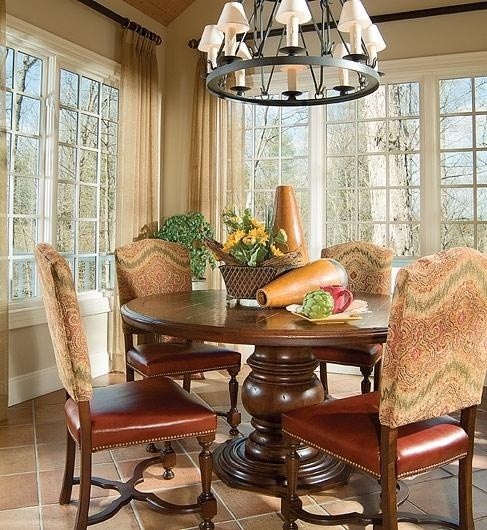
[193,1,389,110]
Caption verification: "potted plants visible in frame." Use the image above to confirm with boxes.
[149,209,216,291]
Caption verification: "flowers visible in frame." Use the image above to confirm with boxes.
[206,207,290,269]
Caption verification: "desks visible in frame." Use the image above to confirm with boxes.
[119,288,392,498]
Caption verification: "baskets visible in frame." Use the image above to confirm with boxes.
[218,265,301,301]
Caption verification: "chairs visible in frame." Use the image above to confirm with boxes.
[113,237,243,453]
[27,241,219,530]
[279,245,487,530]
[312,240,395,401]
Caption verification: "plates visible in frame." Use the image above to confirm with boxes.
[289,311,363,326]
